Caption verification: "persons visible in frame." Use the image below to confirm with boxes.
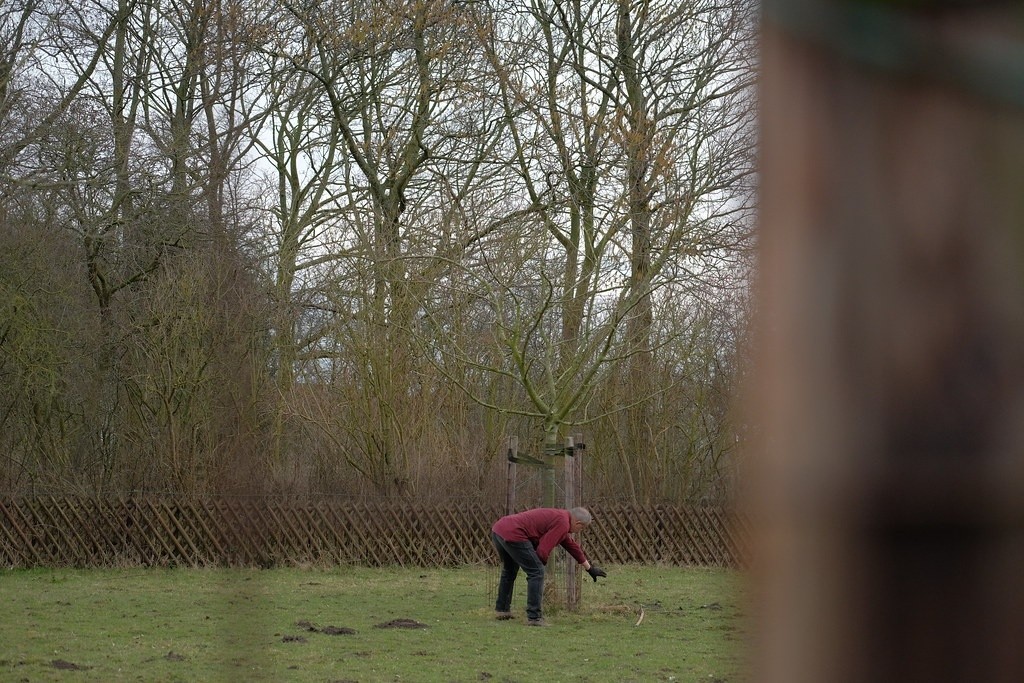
[492,507,608,626]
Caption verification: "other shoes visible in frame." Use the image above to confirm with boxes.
[496,611,517,619]
[527,617,551,627]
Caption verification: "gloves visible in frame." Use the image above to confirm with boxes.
[585,564,607,582]
